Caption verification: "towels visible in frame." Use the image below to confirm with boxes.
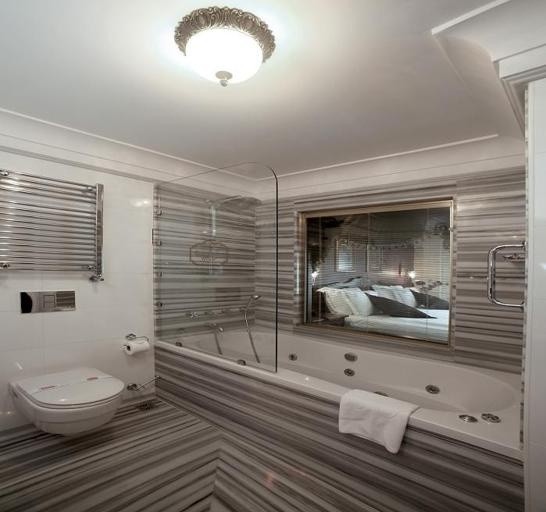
[338,389,420,454]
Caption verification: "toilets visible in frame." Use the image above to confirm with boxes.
[10,365,124,441]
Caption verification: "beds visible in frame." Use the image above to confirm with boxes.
[312,276,449,345]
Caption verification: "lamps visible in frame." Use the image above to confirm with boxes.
[174,6,275,87]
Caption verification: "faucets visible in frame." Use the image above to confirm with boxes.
[206,321,225,335]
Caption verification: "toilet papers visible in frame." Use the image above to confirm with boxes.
[124,337,149,356]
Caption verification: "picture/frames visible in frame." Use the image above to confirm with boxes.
[367,244,383,273]
[336,240,356,272]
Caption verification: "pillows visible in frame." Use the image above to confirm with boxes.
[316,284,449,318]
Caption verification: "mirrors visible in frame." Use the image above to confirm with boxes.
[20,291,75,313]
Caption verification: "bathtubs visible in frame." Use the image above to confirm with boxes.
[154,321,520,464]
